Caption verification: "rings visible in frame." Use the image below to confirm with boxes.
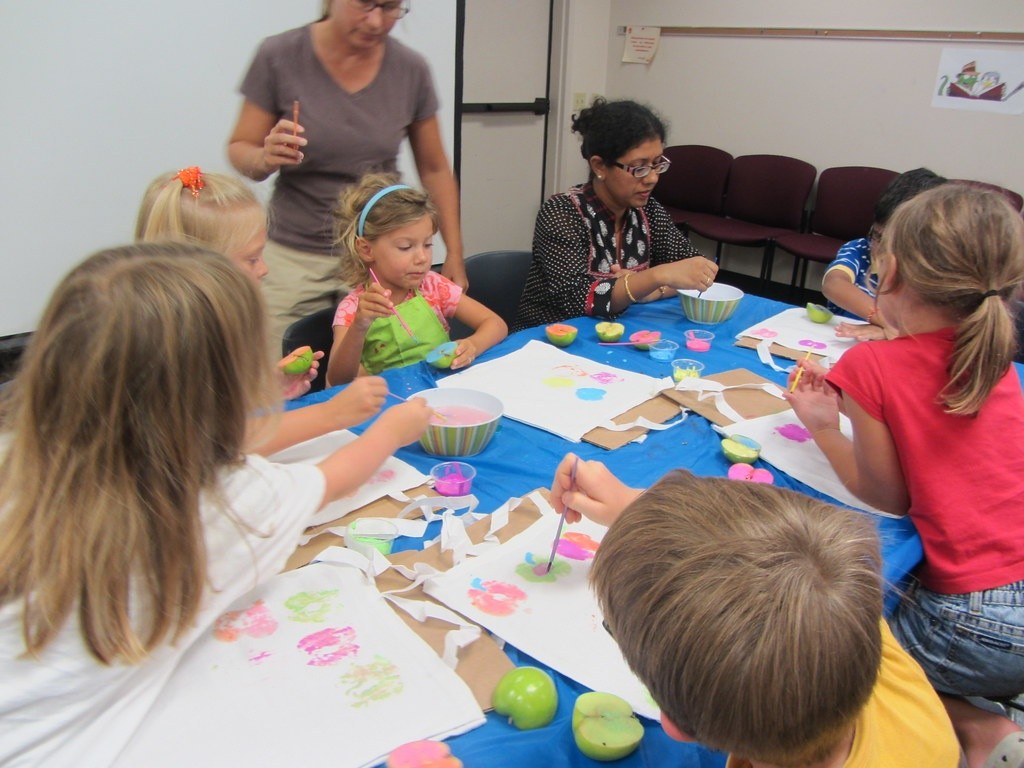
[705,277,710,285]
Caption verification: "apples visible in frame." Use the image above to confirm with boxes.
[728,463,776,485]
[493,667,557,728]
[572,692,643,761]
[282,345,312,372]
[595,322,625,343]
[629,330,662,350]
[426,342,459,369]
[806,303,834,321]
[721,434,759,463]
[546,323,578,345]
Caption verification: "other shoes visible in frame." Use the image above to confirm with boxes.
[984,730,1024,768]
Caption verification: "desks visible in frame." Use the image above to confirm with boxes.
[244,289,1024,768]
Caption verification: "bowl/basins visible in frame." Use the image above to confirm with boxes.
[677,283,744,324]
[406,387,504,457]
[648,340,679,362]
[684,330,715,352]
[430,462,476,496]
[671,359,705,382]
[349,519,398,556]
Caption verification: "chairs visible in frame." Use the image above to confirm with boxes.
[280,252,527,397]
[645,144,1024,308]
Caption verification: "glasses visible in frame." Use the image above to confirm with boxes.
[612,155,672,178]
[867,225,882,248]
[348,0,411,19]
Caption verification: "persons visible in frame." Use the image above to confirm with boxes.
[326,170,508,387]
[819,167,948,342]
[513,96,722,332]
[132,164,389,458]
[781,183,1024,768]
[227,0,471,366]
[0,242,434,768]
[548,451,970,768]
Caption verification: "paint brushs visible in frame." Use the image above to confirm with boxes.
[698,257,718,298]
[790,345,813,393]
[546,457,579,572]
[370,268,419,345]
[598,338,666,346]
[388,391,446,422]
[293,97,300,150]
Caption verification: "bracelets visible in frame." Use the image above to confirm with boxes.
[622,272,638,304]
[867,307,878,325]
[657,287,666,297]
